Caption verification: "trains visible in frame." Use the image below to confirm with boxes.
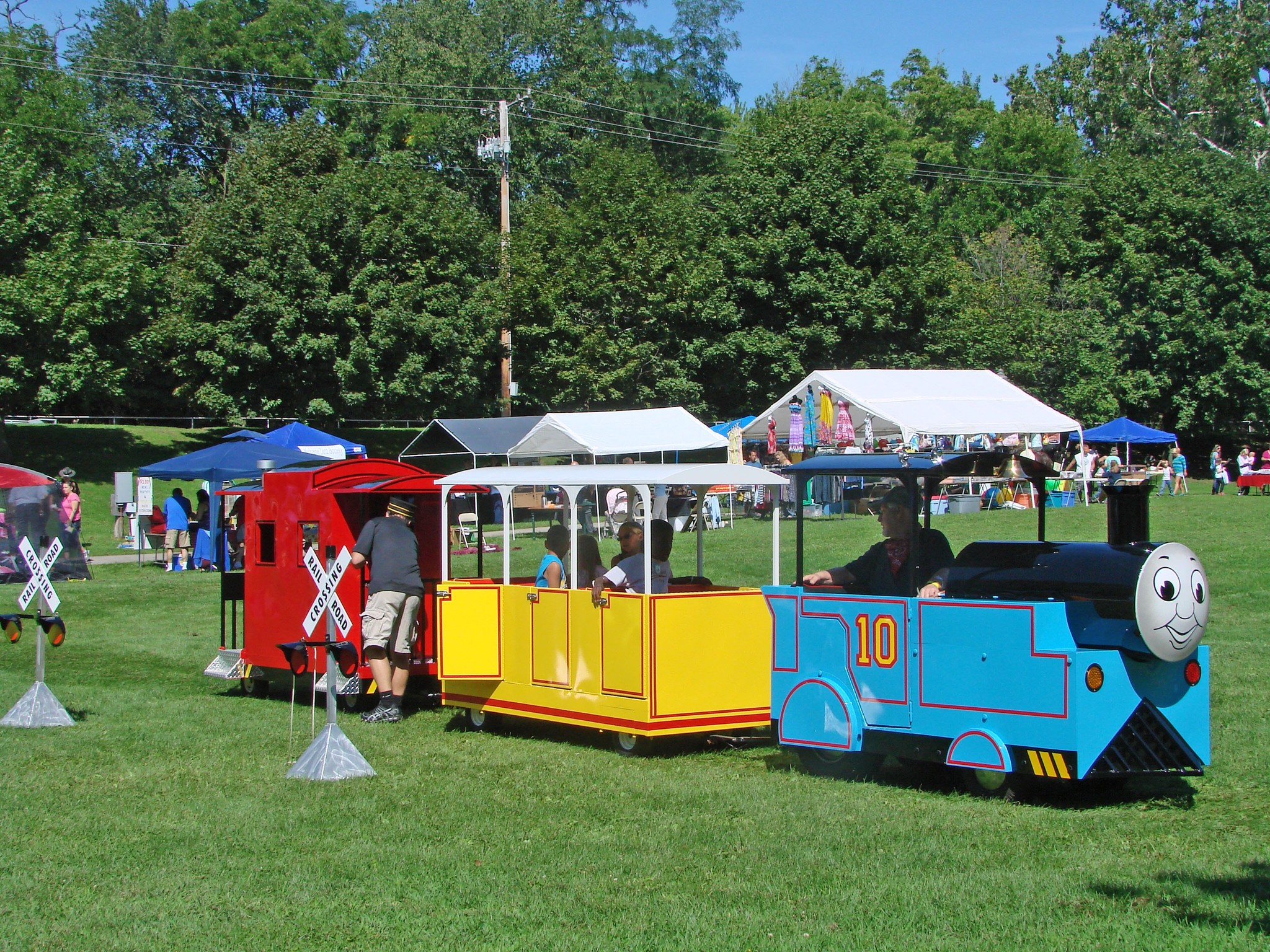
[204,450,1210,804]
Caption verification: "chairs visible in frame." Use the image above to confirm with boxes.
[690,495,716,533]
[603,496,653,538]
[459,512,486,551]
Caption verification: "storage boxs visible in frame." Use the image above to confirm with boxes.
[947,494,981,515]
[1045,489,1075,507]
[922,494,948,515]
[512,486,545,507]
[1012,493,1038,508]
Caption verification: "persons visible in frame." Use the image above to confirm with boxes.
[620,457,642,525]
[8,467,53,555]
[1236,444,1270,496]
[228,488,245,570]
[773,450,792,467]
[569,461,596,533]
[535,519,674,602]
[651,484,670,523]
[164,486,192,573]
[53,479,82,552]
[790,485,955,608]
[743,450,763,474]
[351,496,425,721]
[192,490,220,571]
[1064,444,1189,498]
[1209,444,1228,496]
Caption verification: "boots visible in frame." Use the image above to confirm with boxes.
[181,562,188,570]
[164,563,173,572]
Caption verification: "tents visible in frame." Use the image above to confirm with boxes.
[132,404,795,570]
[1060,416,1189,498]
[707,369,1090,510]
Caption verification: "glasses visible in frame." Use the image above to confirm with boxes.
[617,533,632,541]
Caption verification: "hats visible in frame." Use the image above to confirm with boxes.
[59,466,76,477]
[387,496,417,518]
[1110,447,1118,452]
[867,486,923,514]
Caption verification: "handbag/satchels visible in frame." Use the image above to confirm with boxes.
[1221,470,1229,484]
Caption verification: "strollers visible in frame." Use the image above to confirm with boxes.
[602,488,629,541]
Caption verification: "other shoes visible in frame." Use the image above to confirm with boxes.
[1178,493,1185,496]
[1156,493,1162,497]
[231,561,242,570]
[1168,493,1175,496]
[1210,492,1224,496]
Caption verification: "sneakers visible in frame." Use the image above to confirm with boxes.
[360,703,402,724]
[83,550,90,562]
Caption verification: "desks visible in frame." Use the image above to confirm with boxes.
[1236,475,1270,495]
[528,506,592,540]
[653,497,697,517]
[454,528,484,544]
[142,532,166,565]
[936,469,1174,515]
[705,490,754,518]
